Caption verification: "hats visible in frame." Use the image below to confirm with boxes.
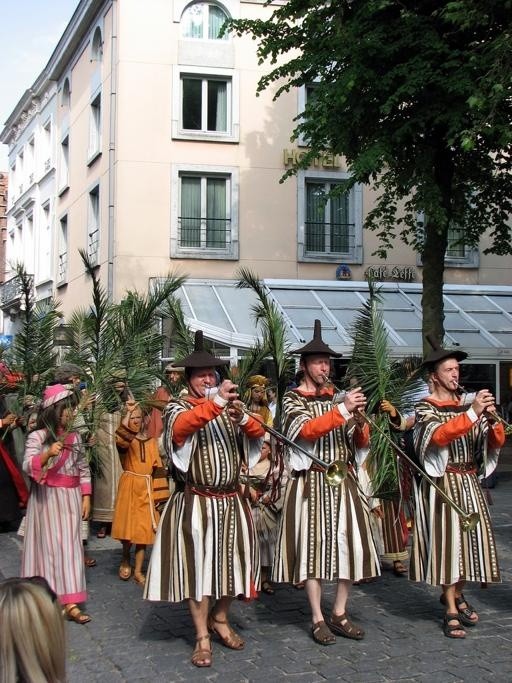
[288,320,342,356]
[419,334,467,366]
[172,330,229,368]
[41,384,74,409]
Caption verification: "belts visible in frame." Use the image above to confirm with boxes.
[189,483,237,496]
[448,462,474,471]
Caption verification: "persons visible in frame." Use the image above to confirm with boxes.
[0,574,69,682]
[2,344,506,668]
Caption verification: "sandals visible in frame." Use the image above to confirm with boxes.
[119,564,145,585]
[262,581,275,594]
[97,527,106,538]
[312,613,364,644]
[440,593,479,638]
[394,560,407,572]
[191,635,213,667]
[66,605,91,624]
[206,607,245,650]
